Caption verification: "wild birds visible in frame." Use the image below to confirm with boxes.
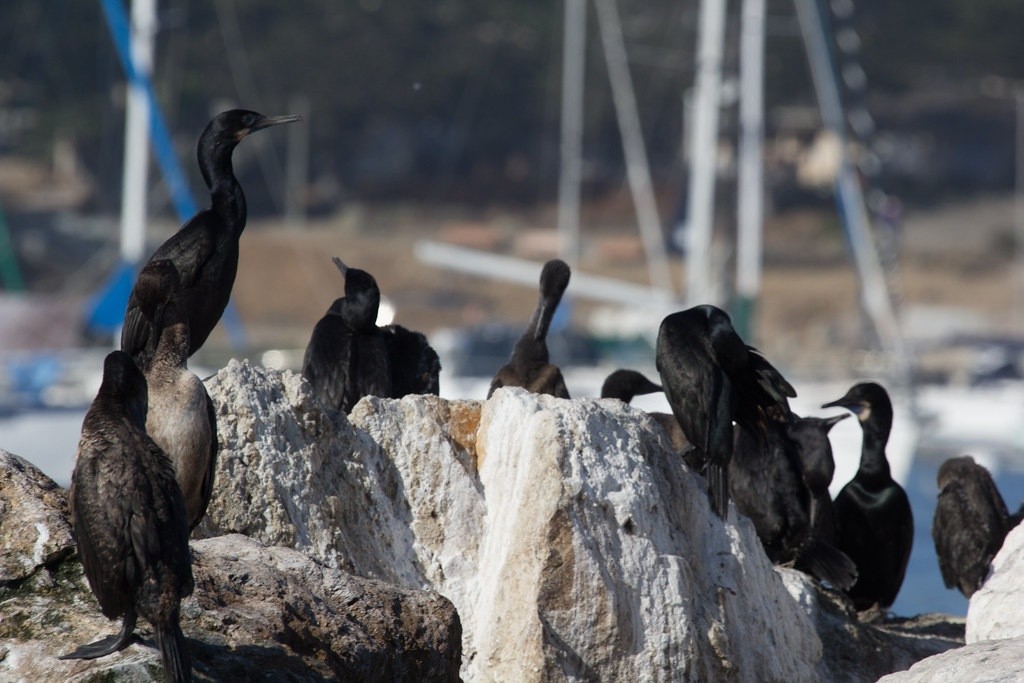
[597,305,916,616]
[481,257,575,401]
[65,107,299,683]
[930,455,1014,600]
[300,260,440,417]
